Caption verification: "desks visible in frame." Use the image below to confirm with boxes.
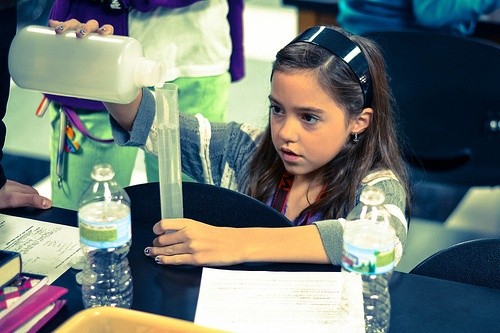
[0,206,500,333]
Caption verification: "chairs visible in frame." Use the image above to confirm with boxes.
[361,31,500,187]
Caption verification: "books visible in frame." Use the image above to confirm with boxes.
[0,249,70,333]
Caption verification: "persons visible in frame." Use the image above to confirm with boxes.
[337,0,500,225]
[50,0,232,214]
[48,19,413,269]
[0,0,52,210]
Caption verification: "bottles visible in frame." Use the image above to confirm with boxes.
[77,164,133,310]
[9,25,166,104]
[341,186,396,333]
